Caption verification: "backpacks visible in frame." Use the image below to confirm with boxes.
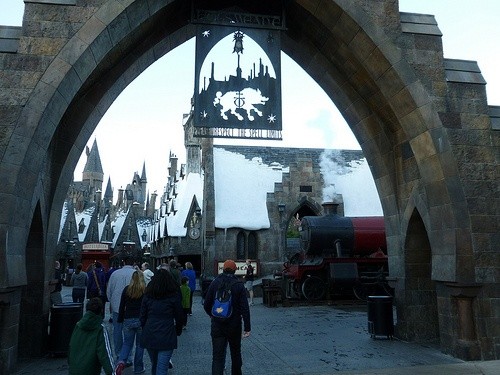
[211,276,244,320]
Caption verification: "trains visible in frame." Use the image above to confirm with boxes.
[282,200,392,306]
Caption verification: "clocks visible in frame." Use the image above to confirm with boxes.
[189,228,200,240]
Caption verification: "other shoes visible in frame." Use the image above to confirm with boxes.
[123,362,132,368]
[169,359,174,369]
[249,301,255,306]
[187,313,193,315]
[113,363,125,375]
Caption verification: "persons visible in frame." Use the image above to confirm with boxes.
[70,263,88,306]
[244,259,254,306]
[114,271,146,375]
[139,269,183,375]
[87,256,196,368]
[67,297,115,375]
[50,261,66,305]
[204,260,251,375]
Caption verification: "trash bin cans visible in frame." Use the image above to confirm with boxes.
[49,301,84,355]
[367,296,395,336]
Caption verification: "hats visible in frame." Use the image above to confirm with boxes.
[223,259,236,270]
